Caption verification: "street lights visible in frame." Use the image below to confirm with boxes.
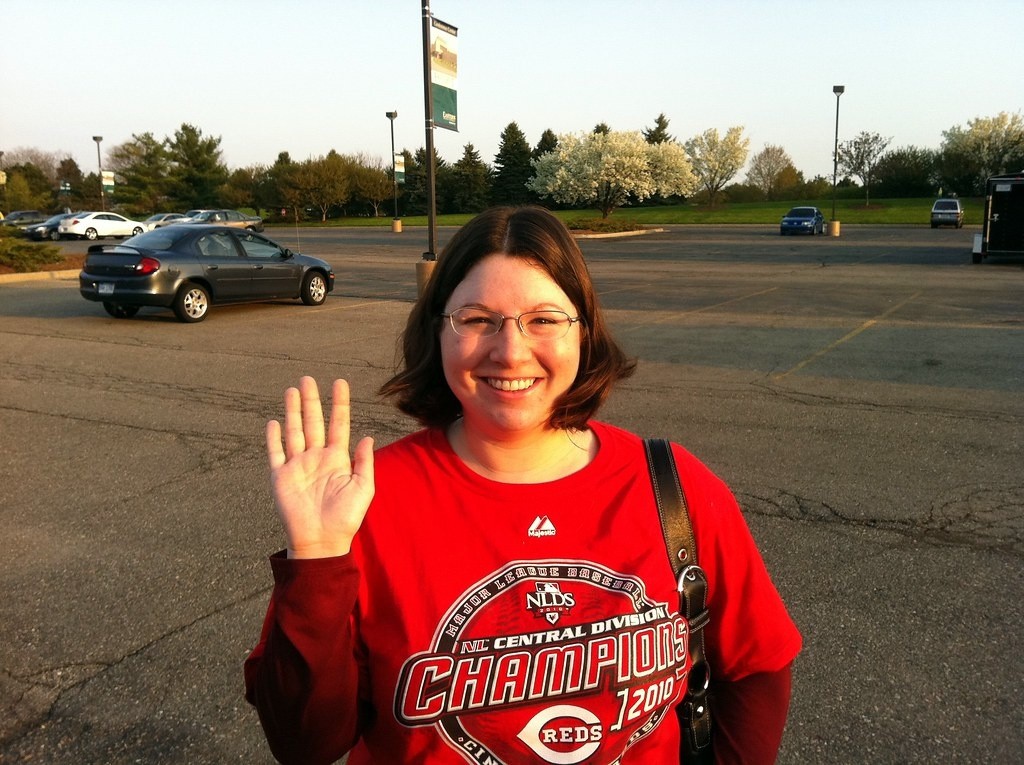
[92,133,106,212]
[827,83,847,236]
[384,109,404,234]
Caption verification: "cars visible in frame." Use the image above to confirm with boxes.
[930,198,964,229]
[780,205,825,236]
[0,208,265,241]
[76,222,339,324]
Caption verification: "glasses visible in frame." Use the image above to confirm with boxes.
[441,302,578,340]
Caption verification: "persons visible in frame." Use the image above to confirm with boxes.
[243,206,803,765]
